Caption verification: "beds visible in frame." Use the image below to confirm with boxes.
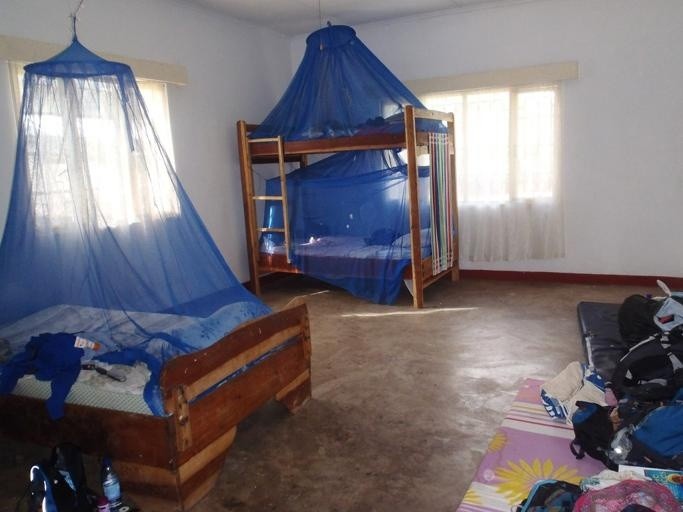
[0,296,309,511]
[236,105,459,309]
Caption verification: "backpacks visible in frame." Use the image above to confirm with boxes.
[17,438,94,512]
[631,389,683,470]
[612,331,683,400]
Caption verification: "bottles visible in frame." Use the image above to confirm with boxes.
[101,460,132,512]
[98,497,110,512]
